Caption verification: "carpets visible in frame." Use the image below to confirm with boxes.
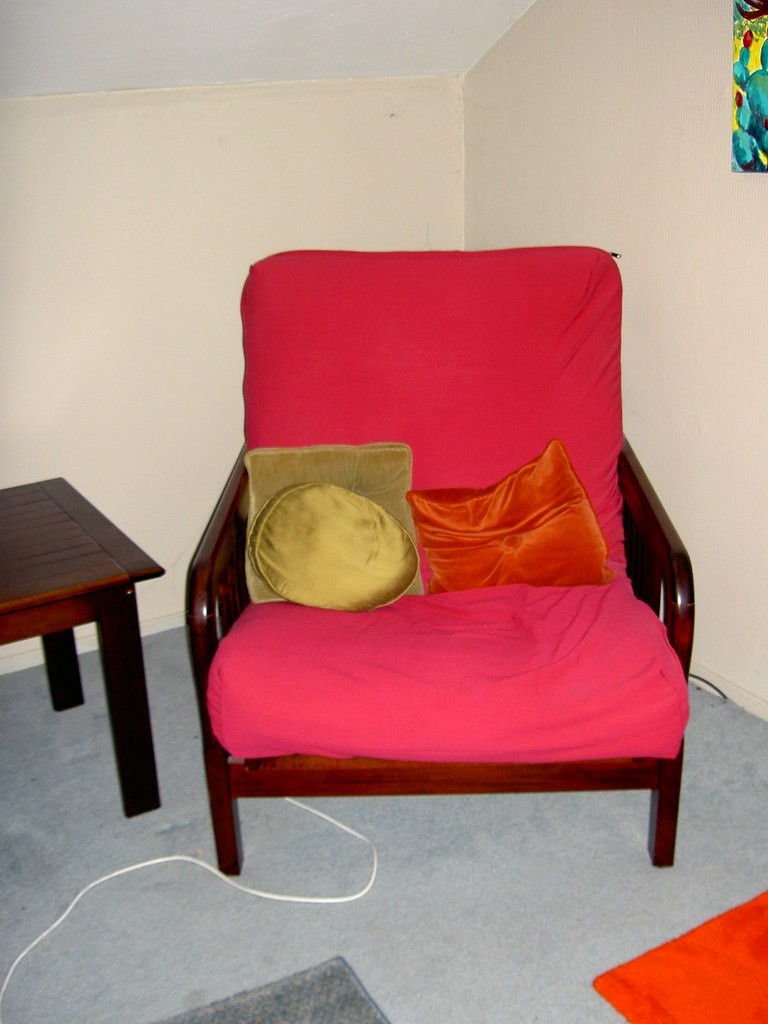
[153,953,392,1024]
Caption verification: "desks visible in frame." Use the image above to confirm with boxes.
[592,890,768,1024]
[0,477,166,819]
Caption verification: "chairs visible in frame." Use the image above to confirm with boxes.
[184,247,695,876]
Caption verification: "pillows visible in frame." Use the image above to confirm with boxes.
[248,482,419,612]
[405,440,617,591]
[242,442,423,605]
